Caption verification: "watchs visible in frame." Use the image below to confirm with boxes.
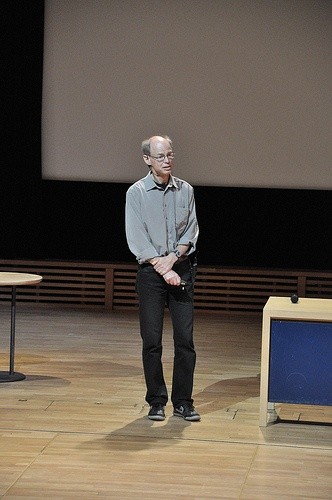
[172,249,182,259]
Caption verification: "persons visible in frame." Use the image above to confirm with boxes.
[125,135,201,421]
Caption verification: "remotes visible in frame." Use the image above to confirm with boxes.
[181,281,191,286]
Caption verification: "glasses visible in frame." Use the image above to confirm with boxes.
[149,152,175,161]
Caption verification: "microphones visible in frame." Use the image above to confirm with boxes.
[291,294,298,303]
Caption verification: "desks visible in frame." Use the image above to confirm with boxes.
[258,296,332,427]
[0,272,43,382]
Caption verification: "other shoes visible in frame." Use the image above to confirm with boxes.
[173,404,201,421]
[148,404,165,420]
